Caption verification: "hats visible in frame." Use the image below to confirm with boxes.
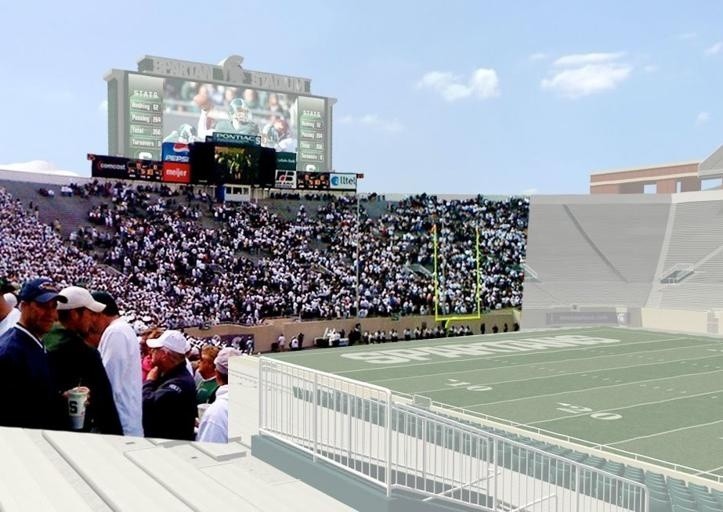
[211,346,241,375]
[55,284,106,316]
[143,328,192,357]
[16,274,67,306]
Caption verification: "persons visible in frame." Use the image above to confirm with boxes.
[0,355,229,443]
[0,178,529,356]
[163,78,297,156]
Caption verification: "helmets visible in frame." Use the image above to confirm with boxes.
[227,98,249,121]
[268,113,286,131]
[177,124,197,144]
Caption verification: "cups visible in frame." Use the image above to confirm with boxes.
[67,387,91,430]
